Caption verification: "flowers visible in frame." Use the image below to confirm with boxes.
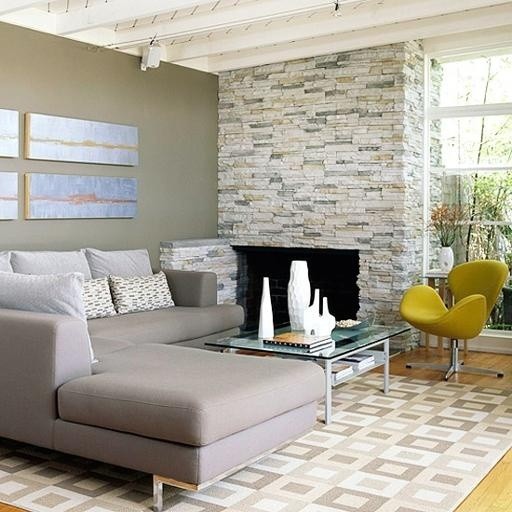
[429,198,472,246]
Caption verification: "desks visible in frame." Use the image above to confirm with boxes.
[424,273,468,358]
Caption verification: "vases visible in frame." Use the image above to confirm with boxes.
[258,276,277,341]
[438,245,456,274]
[287,259,314,332]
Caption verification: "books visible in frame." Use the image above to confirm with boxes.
[263,341,333,353]
[323,353,375,381]
[262,332,332,349]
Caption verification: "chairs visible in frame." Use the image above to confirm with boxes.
[398,259,509,381]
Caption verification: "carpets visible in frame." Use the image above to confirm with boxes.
[2,370,512,512]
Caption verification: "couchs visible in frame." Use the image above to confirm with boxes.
[2,269,326,512]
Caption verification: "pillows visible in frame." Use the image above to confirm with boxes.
[108,270,176,314]
[11,242,94,282]
[3,270,104,365]
[83,276,118,320]
[85,244,156,279]
